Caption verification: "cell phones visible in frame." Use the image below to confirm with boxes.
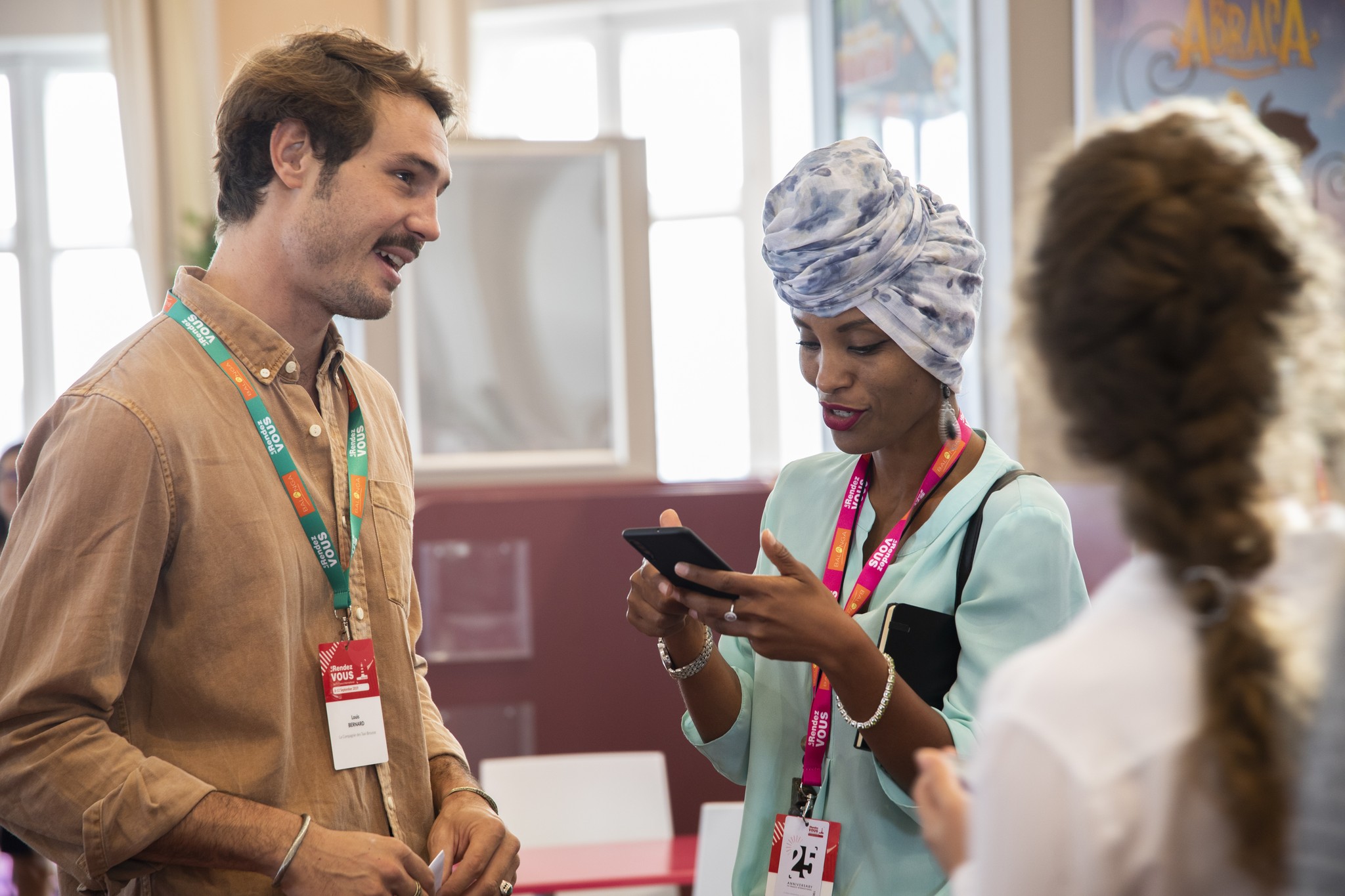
[621,525,740,600]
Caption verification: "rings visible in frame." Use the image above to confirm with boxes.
[500,880,513,896]
[413,879,422,896]
[724,602,739,623]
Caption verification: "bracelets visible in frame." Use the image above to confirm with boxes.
[434,786,501,817]
[834,652,897,730]
[270,812,314,890]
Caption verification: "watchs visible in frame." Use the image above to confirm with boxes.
[657,622,714,680]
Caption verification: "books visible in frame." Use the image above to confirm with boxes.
[855,602,960,753]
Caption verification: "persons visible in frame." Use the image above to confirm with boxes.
[909,91,1345,896]
[623,139,1091,895]
[0,444,81,896]
[0,31,522,896]
